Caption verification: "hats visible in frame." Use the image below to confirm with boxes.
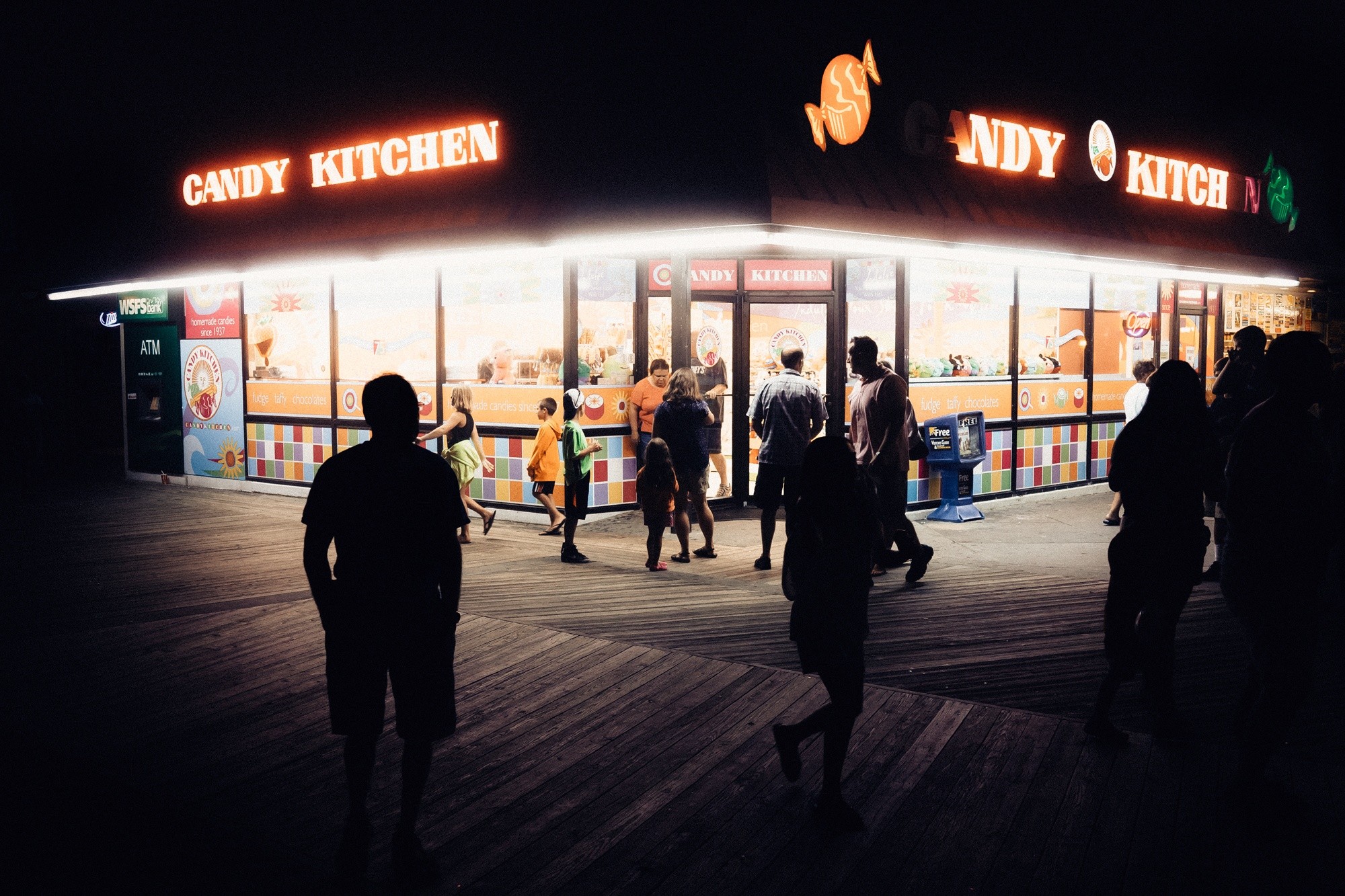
[563,388,585,420]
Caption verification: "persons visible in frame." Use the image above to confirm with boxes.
[412,383,498,544]
[745,345,830,569]
[1086,322,1345,759]
[628,358,678,531]
[525,398,568,536]
[686,329,732,500]
[298,374,474,872]
[864,362,921,576]
[636,438,679,570]
[771,436,883,829]
[846,336,936,584]
[559,388,604,563]
[652,366,717,564]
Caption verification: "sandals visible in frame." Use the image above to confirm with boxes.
[671,553,690,563]
[693,546,718,557]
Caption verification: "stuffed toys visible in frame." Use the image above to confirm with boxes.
[476,327,636,386]
[751,349,1067,392]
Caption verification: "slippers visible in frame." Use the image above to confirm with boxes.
[483,510,496,535]
[1103,518,1121,525]
[547,518,568,535]
[538,529,562,535]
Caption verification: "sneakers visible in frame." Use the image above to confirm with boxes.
[905,544,934,583]
[561,542,590,563]
[714,482,731,499]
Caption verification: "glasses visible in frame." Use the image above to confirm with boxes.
[414,402,424,411]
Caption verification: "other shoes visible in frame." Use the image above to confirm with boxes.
[645,560,667,571]
[340,816,375,849]
[813,796,865,833]
[390,831,426,863]
[774,723,802,783]
